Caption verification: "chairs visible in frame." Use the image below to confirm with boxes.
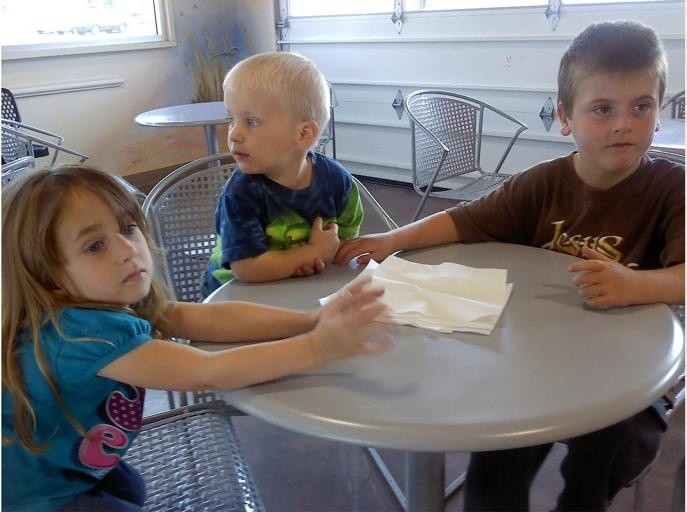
[2,156,32,187]
[122,403,263,510]
[141,154,398,416]
[406,90,531,226]
[3,87,89,172]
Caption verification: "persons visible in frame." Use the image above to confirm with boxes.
[333,20,686,510]
[1,165,392,512]
[197,51,364,304]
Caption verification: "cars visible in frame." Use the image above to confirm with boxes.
[36,10,133,37]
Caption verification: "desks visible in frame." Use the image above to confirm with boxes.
[135,100,231,213]
[189,241,685,512]
